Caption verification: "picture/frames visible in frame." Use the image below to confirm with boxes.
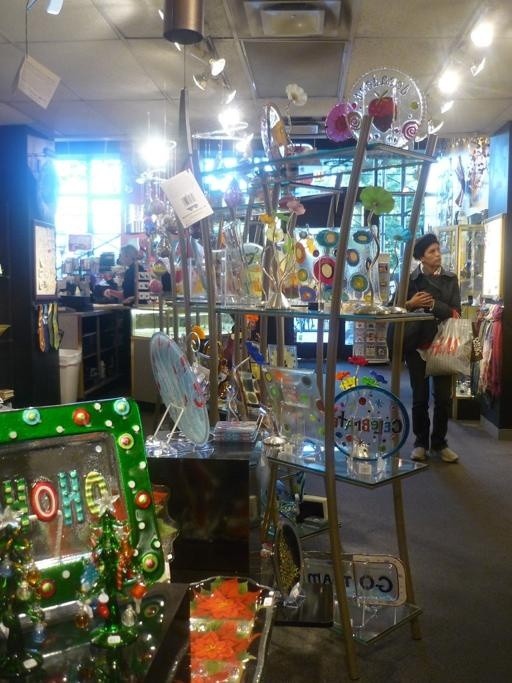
[31,218,58,300]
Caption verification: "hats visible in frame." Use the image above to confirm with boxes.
[412,234,439,260]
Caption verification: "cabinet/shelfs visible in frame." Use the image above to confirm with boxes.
[161,114,438,681]
[55,247,213,401]
[147,428,269,592]
[0,580,194,681]
[435,224,484,305]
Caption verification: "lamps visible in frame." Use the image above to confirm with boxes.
[414,12,498,143]
[158,7,254,152]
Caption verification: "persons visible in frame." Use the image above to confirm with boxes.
[102,244,149,306]
[402,233,461,463]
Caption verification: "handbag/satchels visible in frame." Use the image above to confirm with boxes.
[472,337,483,362]
[424,308,473,377]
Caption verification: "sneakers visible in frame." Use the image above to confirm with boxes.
[429,448,458,462]
[411,447,426,460]
